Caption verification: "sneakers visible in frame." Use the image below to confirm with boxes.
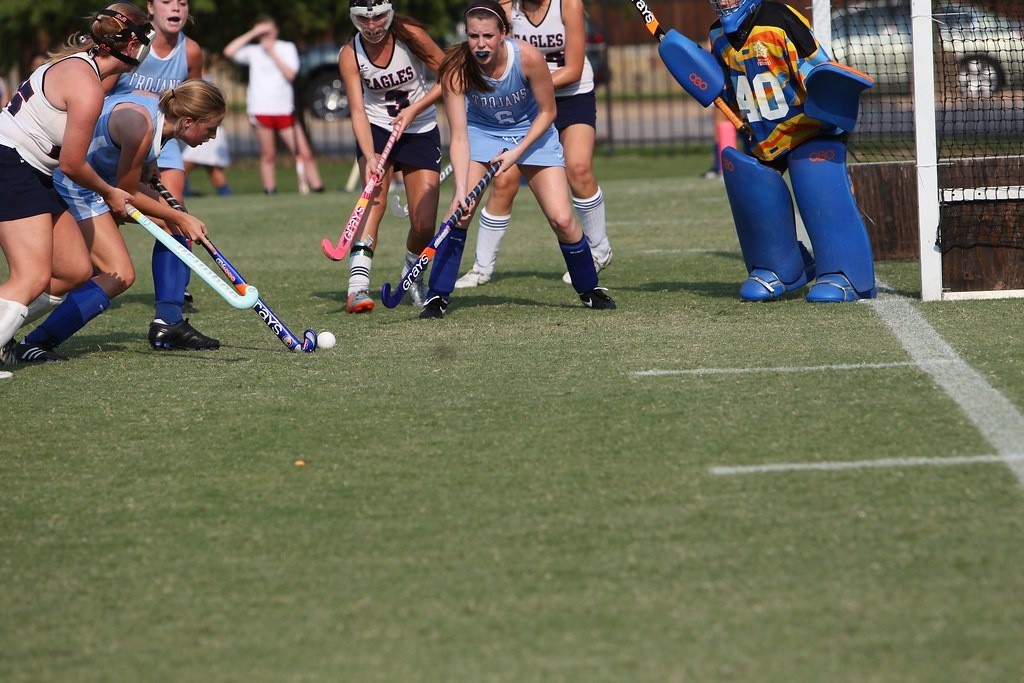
[346,290,374,314]
[14,342,68,363]
[578,286,616,310]
[562,247,613,285]
[149,318,220,350]
[454,270,491,288]
[420,297,447,319]
[0,338,19,366]
[410,279,427,306]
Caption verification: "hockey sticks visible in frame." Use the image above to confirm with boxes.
[152,172,318,354]
[124,202,260,310]
[630,0,758,144]
[321,120,402,261]
[381,146,509,309]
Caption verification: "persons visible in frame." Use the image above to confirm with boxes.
[419,0,617,319]
[700,0,878,301]
[0,0,232,380]
[453,0,615,289]
[223,14,323,195]
[337,0,447,313]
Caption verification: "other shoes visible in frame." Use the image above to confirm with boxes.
[181,292,197,312]
[738,266,814,300]
[806,273,877,301]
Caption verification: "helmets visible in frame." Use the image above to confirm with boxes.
[709,0,761,33]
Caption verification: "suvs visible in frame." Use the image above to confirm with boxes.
[293,10,611,125]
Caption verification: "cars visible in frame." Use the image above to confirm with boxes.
[808,0,1024,98]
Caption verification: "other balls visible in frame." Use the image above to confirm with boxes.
[317,332,336,350]
